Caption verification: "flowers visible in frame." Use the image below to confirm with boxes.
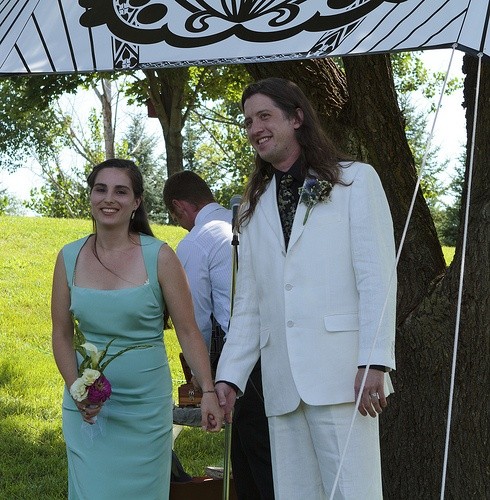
[68,313,152,421]
[296,179,333,226]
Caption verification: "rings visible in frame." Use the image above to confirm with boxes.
[81,406,86,414]
[371,390,380,401]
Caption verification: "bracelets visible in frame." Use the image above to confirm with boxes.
[200,390,217,395]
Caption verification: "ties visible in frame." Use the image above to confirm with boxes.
[278,174,297,251]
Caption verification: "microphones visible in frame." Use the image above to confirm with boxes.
[230,194,243,245]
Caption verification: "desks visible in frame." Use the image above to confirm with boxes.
[173,407,202,427]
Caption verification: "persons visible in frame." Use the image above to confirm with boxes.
[49,157,226,500]
[209,77,398,500]
[160,168,276,500]
